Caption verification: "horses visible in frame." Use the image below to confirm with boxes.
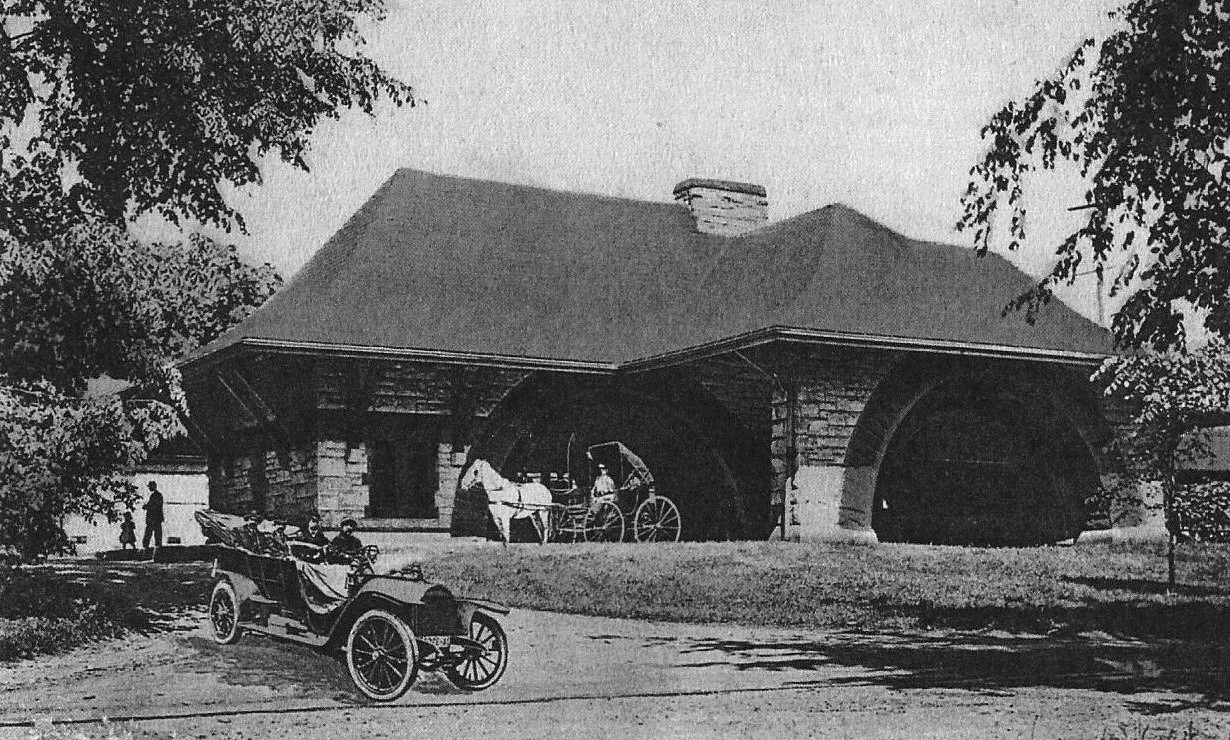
[461,458,557,551]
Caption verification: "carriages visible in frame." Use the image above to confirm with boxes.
[460,441,682,546]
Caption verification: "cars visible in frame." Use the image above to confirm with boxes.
[194,510,511,701]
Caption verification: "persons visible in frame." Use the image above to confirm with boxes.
[239,509,365,563]
[591,463,617,505]
[119,511,137,552]
[142,481,165,549]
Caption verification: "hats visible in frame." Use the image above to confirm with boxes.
[340,516,356,527]
[146,481,156,486]
[598,463,609,469]
